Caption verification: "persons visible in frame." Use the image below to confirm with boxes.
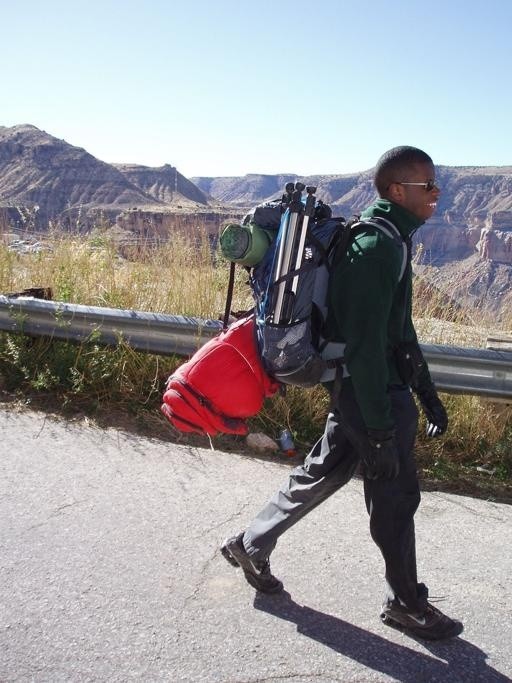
[221,146,464,641]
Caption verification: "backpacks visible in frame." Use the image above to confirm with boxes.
[248,193,410,391]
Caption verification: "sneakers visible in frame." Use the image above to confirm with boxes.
[220,531,286,594]
[379,585,464,640]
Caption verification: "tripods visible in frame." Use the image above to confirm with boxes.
[269,181,318,330]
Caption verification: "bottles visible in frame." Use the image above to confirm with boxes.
[278,421,297,457]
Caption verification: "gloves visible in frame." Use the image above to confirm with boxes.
[415,384,448,437]
[369,424,403,480]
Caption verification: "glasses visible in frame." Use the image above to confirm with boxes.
[395,177,439,192]
